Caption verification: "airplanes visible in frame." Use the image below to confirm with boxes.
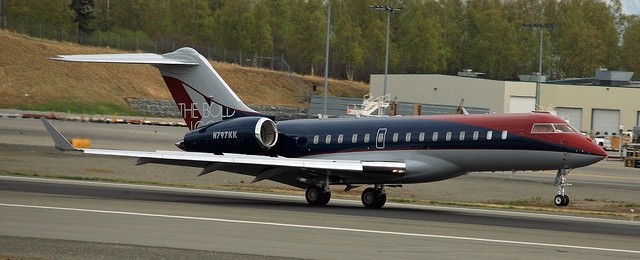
[40,47,607,208]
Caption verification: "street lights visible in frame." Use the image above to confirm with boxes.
[521,23,553,111]
[368,4,401,114]
